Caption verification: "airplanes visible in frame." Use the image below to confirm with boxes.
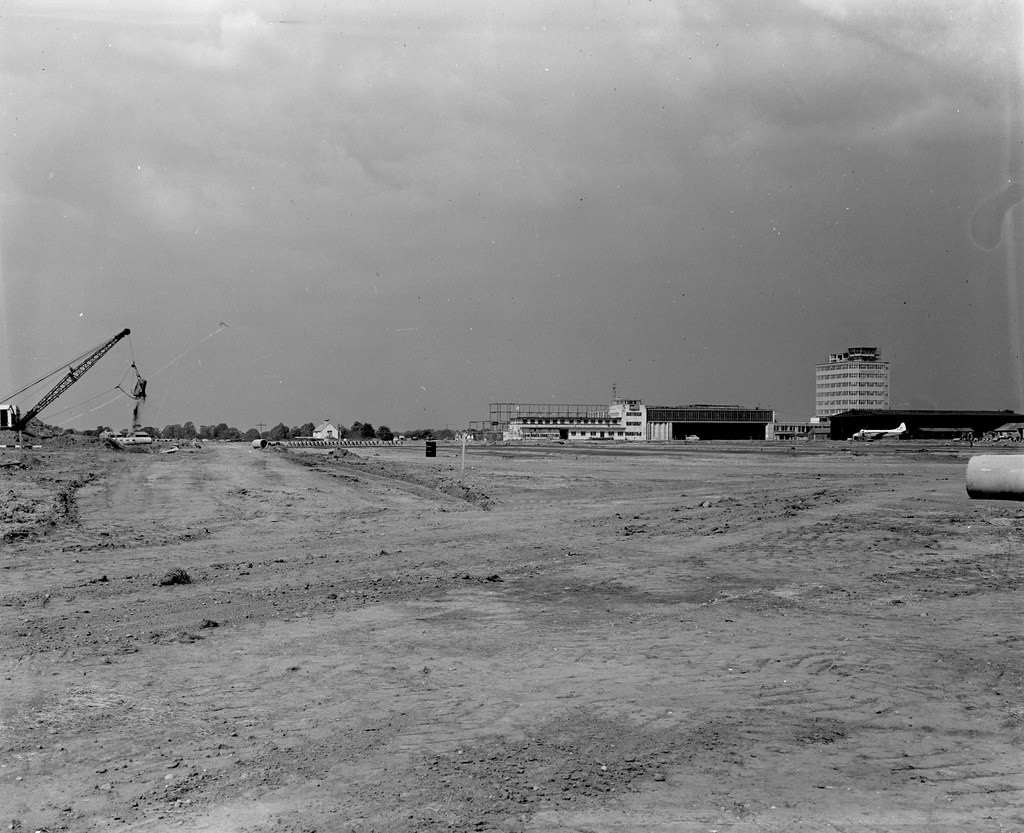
[852,422,907,442]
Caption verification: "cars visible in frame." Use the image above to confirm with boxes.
[686,435,699,441]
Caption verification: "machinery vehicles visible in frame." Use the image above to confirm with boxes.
[0,327,148,433]
